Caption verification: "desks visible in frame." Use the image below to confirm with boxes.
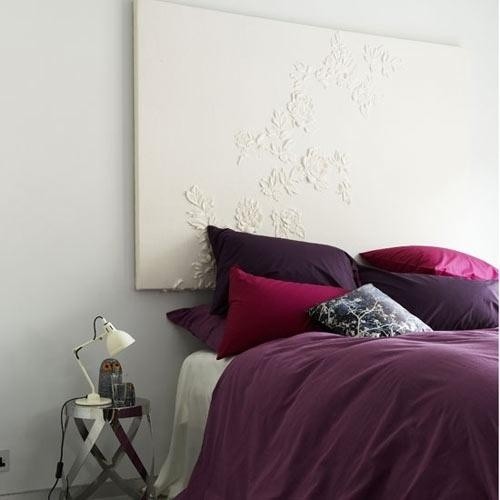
[61,390,153,500]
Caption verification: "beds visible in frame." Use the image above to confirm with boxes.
[158,228,498,500]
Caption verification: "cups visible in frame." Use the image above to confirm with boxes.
[110,372,127,407]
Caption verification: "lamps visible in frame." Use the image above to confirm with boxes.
[71,315,137,406]
